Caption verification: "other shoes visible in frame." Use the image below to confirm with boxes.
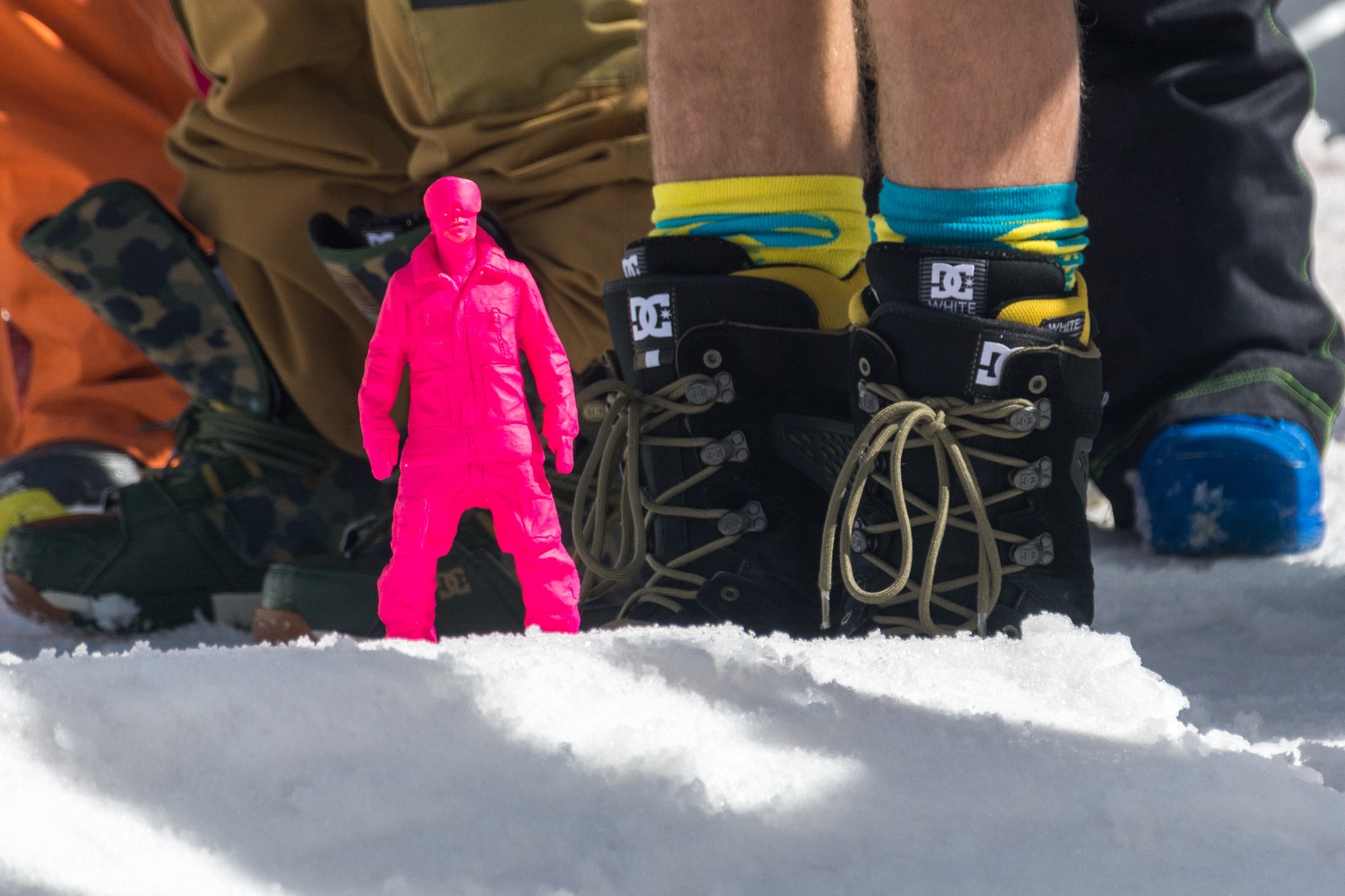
[583,235,854,641]
[1133,414,1326,557]
[831,241,1104,634]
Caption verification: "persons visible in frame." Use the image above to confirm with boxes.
[0,0,1345,650]
[357,177,585,643]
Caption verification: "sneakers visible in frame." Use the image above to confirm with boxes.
[252,210,623,645]
[3,175,390,635]
[0,438,143,541]
